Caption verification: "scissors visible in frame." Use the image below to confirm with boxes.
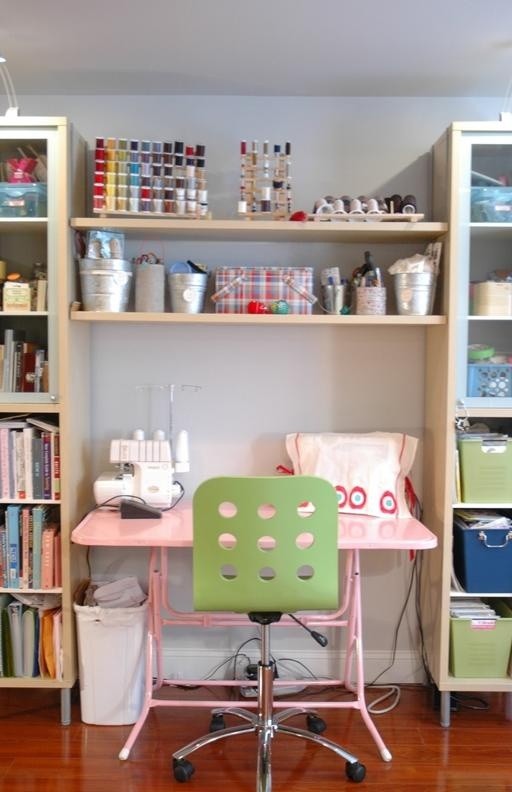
[135,253,161,263]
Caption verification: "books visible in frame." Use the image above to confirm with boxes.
[1,326,62,679]
[450,423,512,621]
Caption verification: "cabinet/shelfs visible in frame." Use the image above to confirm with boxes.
[0,114,88,727]
[71,215,449,324]
[420,119,512,729]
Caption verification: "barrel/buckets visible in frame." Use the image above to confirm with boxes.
[77,254,134,313]
[167,272,211,316]
[391,271,439,316]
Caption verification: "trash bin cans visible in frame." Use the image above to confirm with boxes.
[72,579,147,726]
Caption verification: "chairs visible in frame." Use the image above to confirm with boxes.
[173,477,367,792]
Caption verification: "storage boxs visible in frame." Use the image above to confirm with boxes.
[0,183,47,216]
[469,281,512,314]
[211,266,318,314]
[457,438,512,504]
[454,521,512,593]
[451,600,512,677]
[470,186,512,224]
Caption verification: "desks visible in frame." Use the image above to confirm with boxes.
[69,506,439,762]
